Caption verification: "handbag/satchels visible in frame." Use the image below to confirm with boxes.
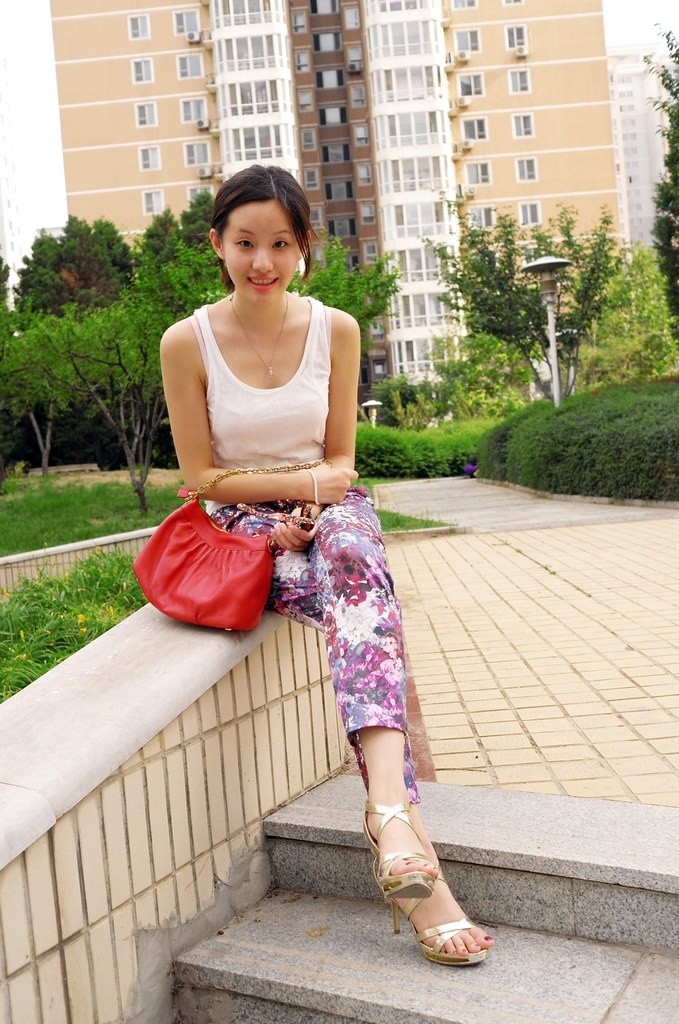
[132,495,275,631]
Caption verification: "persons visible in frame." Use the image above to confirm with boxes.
[159,164,496,966]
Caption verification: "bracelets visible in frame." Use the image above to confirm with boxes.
[307,470,321,505]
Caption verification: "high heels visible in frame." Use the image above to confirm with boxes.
[362,800,436,935]
[390,859,487,965]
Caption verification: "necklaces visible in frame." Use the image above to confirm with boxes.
[230,291,288,376]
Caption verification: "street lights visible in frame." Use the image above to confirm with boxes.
[360,398,384,430]
[517,254,573,409]
[556,328,583,399]
